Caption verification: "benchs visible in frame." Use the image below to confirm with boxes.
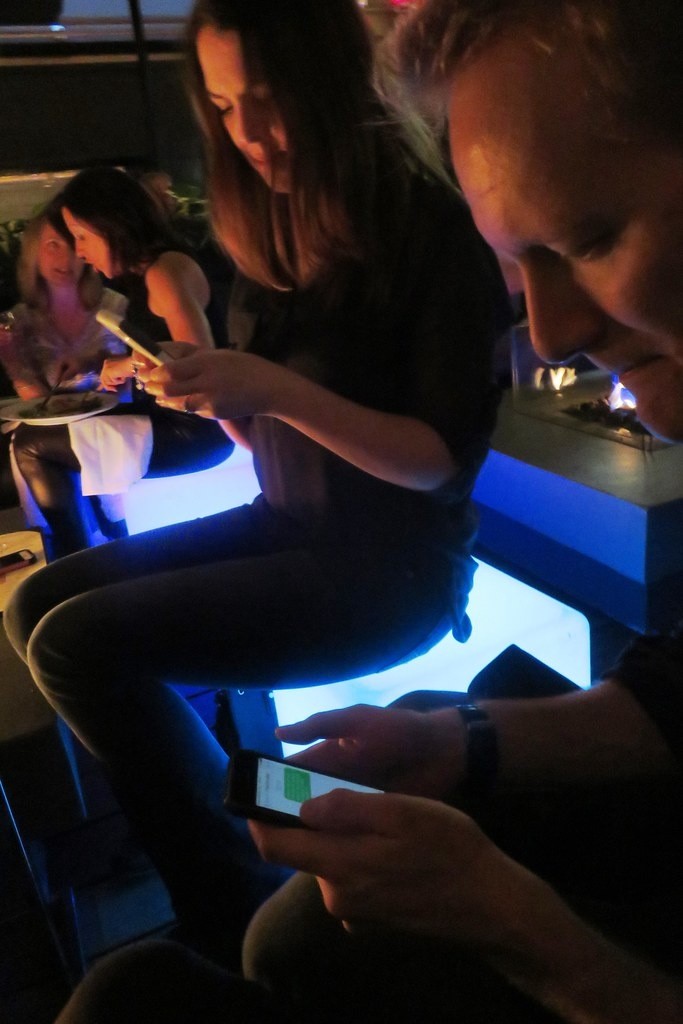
[122,437,593,779]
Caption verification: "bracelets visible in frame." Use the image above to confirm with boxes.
[129,358,144,391]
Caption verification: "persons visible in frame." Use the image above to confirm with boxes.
[47,935,282,1023]
[3,0,514,978]
[235,0,683,1024]
[12,165,234,558]
[0,205,130,565]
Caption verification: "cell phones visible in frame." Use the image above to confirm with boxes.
[96,311,175,368]
[224,750,389,831]
[0,548,37,577]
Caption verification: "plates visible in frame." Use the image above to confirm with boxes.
[2,393,120,427]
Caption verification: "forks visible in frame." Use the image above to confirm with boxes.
[31,363,70,416]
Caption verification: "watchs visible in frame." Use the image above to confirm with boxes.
[450,700,506,801]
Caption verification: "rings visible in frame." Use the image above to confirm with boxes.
[184,394,193,414]
